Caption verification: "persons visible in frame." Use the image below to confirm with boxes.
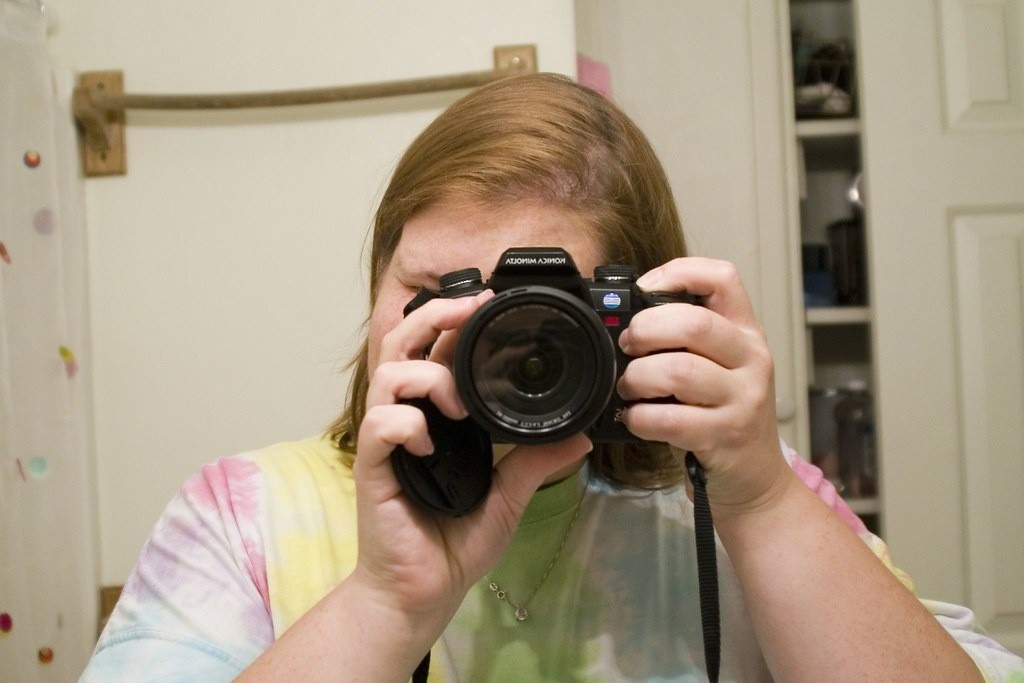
[80,73,1023,683]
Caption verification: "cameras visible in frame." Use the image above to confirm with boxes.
[419,247,700,441]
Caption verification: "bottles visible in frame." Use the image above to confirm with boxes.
[807,379,877,523]
[802,244,838,310]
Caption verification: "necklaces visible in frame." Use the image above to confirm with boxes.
[484,478,590,622]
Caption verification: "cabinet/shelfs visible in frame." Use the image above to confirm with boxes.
[780,0,880,531]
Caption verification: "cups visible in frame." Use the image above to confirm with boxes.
[829,218,865,307]
